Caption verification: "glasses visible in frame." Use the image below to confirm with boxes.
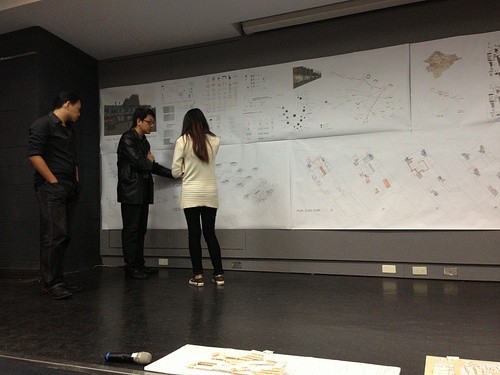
[144,120,154,125]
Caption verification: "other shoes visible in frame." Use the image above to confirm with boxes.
[127,270,149,279]
[143,268,159,274]
[47,287,72,299]
[64,284,83,292]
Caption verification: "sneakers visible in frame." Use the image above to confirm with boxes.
[211,275,224,285]
[188,273,204,286]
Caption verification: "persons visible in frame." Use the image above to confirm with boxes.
[116,106,183,279]
[171,108,225,287]
[28,91,82,299]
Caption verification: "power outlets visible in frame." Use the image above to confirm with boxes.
[444,268,458,275]
[231,262,242,268]
[381,265,396,274]
[413,267,427,275]
[158,259,168,265]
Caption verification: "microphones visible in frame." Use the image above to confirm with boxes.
[104,351,152,365]
